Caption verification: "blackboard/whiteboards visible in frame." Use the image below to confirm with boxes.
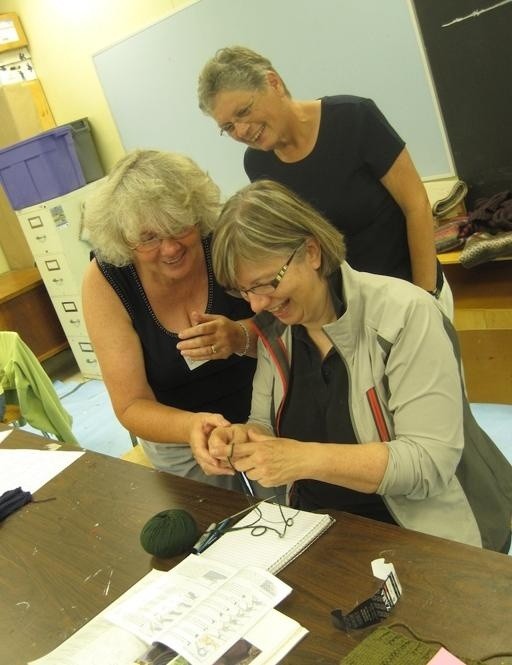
[92,0,456,203]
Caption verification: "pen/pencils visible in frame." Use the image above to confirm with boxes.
[192,519,227,552]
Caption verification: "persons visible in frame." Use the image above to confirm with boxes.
[81,148,286,508]
[208,179,511,558]
[195,44,468,399]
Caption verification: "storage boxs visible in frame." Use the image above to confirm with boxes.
[0,118,106,210]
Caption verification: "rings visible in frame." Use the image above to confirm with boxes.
[211,345,218,353]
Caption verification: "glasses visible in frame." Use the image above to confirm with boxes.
[119,222,201,253]
[218,250,301,302]
[214,89,262,138]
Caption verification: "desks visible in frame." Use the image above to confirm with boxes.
[1,269,72,364]
[1,422,510,665]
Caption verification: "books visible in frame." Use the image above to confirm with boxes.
[198,502,336,576]
[100,552,293,665]
[24,567,311,665]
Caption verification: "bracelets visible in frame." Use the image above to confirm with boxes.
[233,320,250,358]
[427,288,438,296]
